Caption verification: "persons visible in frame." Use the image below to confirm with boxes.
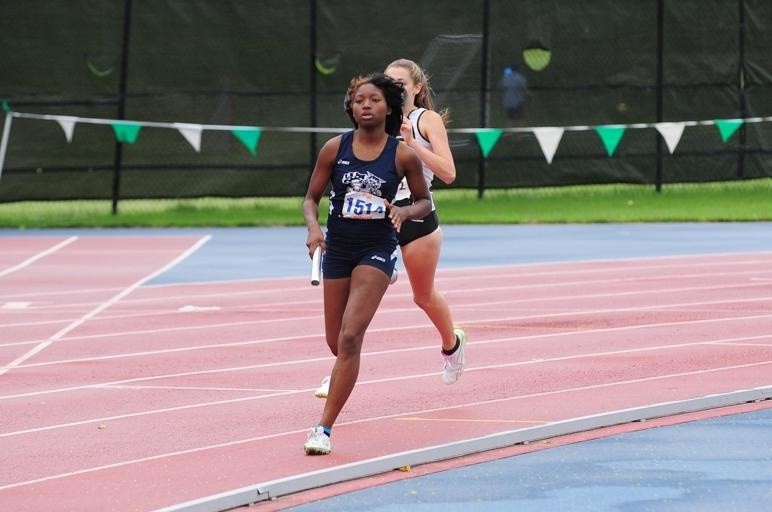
[302,72,432,456]
[314,58,468,400]
[501,61,533,128]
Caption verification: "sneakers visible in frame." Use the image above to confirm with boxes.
[303,425,332,455]
[440,328,466,385]
[315,376,331,398]
